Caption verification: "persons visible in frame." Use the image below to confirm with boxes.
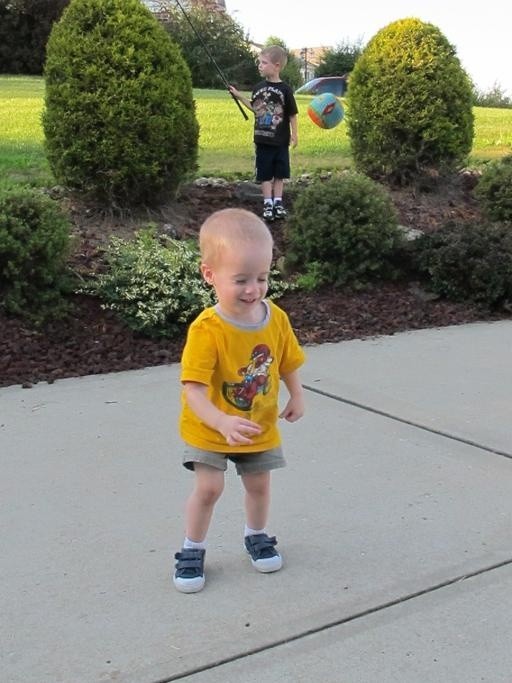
[229,46,298,222]
[174,208,305,593]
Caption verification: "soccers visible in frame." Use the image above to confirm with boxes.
[307,93,343,129]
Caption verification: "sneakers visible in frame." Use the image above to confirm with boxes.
[242,531,283,574]
[262,201,288,222]
[174,546,206,593]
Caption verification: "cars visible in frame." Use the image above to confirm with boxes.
[293,77,347,98]
